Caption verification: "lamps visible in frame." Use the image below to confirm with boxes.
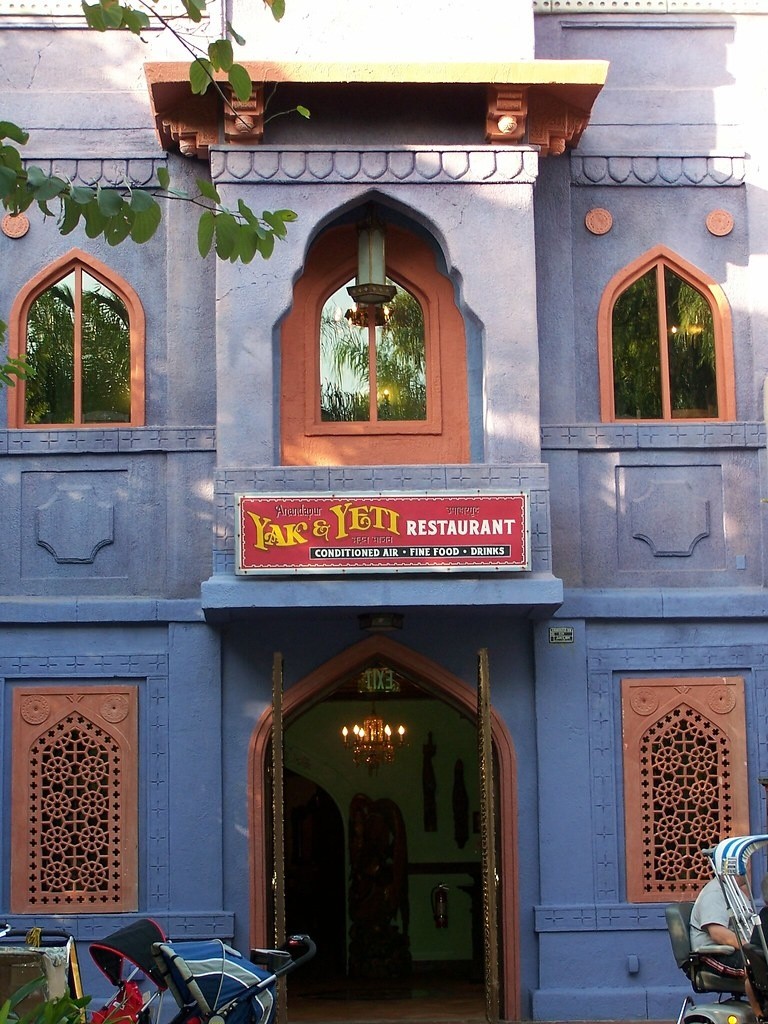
[344,302,397,327]
[342,703,409,771]
[346,220,397,303]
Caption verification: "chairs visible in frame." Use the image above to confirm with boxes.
[664,903,753,994]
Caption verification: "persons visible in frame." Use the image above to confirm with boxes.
[690,874,768,996]
[348,855,414,998]
[750,879,768,945]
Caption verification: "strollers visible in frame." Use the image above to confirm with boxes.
[151,934,317,1024]
[88,917,169,1023]
